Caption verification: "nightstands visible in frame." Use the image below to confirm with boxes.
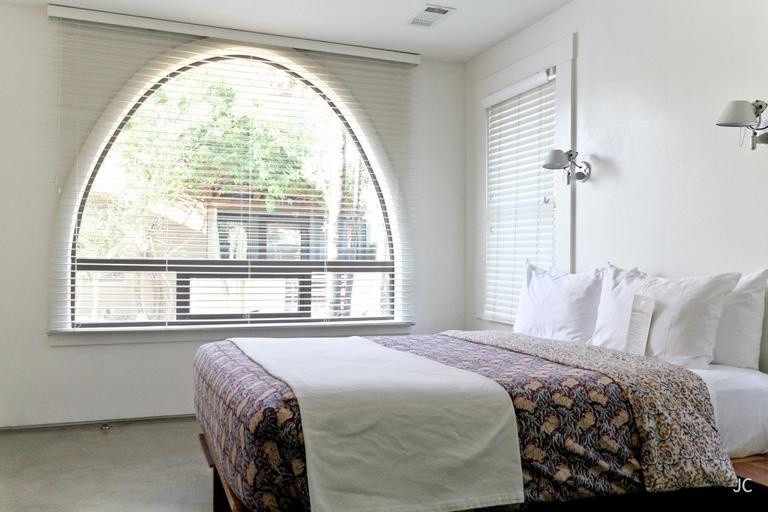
[728,456,768,490]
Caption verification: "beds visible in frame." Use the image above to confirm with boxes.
[193,255,768,512]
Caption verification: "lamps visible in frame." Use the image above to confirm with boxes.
[718,99,768,151]
[541,148,591,182]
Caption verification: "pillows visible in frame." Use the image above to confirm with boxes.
[714,269,768,371]
[513,258,636,351]
[586,262,744,369]
